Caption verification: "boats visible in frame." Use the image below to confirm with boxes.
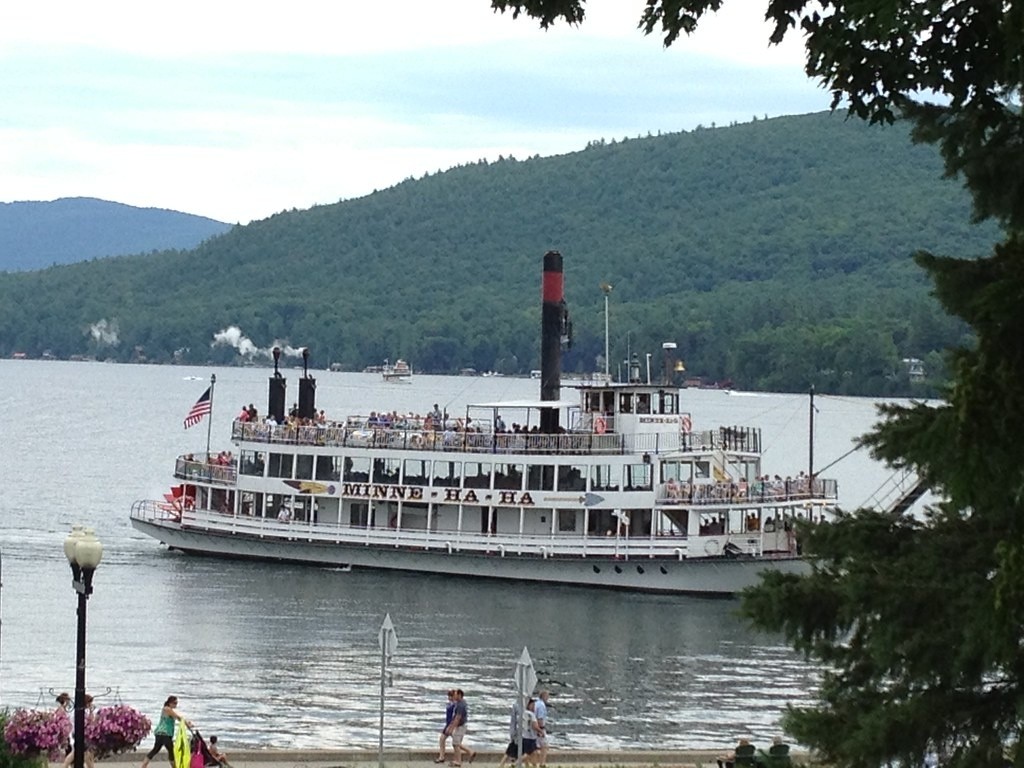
[127,250,839,596]
[381,357,412,384]
[529,369,541,380]
[481,370,504,377]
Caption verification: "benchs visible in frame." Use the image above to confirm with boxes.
[720,744,791,768]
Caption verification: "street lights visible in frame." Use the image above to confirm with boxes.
[61,524,102,768]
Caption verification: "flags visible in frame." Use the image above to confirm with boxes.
[184,386,211,429]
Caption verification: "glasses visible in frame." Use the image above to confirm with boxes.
[171,702,177,704]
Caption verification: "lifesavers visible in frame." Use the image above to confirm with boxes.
[682,418,693,433]
[595,418,607,434]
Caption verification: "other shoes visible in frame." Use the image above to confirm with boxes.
[449,761,461,767]
[470,752,476,763]
[435,758,444,763]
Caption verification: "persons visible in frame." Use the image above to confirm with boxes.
[238,403,573,458]
[745,513,759,531]
[637,396,649,413]
[494,465,581,491]
[766,513,829,532]
[46,691,100,768]
[141,695,192,768]
[219,497,234,514]
[719,735,783,768]
[187,450,264,476]
[278,505,289,522]
[434,689,477,768]
[699,517,720,536]
[209,736,233,768]
[665,471,816,504]
[669,530,675,536]
[609,400,614,415]
[498,690,549,768]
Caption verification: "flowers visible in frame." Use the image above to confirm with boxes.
[82,698,150,761]
[2,707,73,762]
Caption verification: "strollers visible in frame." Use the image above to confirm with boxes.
[188,724,227,768]
